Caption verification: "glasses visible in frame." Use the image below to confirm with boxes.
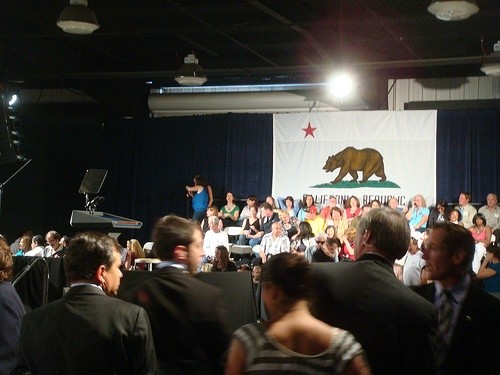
[316,240,325,244]
[47,240,55,244]
[437,207,444,210]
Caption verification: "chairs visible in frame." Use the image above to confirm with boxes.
[224,226,251,260]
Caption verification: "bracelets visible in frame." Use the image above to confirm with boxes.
[416,227,419,229]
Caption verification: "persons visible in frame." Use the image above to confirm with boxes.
[201,205,223,238]
[0,235,26,375]
[253,222,291,267]
[476,242,500,299]
[186,176,213,224]
[9,231,156,272]
[219,192,239,243]
[478,193,500,246]
[196,194,500,287]
[224,253,373,375]
[256,209,439,375]
[120,215,233,375]
[15,231,159,375]
[406,222,500,375]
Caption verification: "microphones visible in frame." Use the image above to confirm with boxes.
[85,196,104,208]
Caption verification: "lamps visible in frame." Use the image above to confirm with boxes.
[480,59,500,76]
[1,91,17,105]
[57,0,100,34]
[427,0,479,22]
[175,52,207,86]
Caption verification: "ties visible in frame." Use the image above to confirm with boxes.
[438,291,452,347]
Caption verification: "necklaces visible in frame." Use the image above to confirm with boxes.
[476,226,482,234]
